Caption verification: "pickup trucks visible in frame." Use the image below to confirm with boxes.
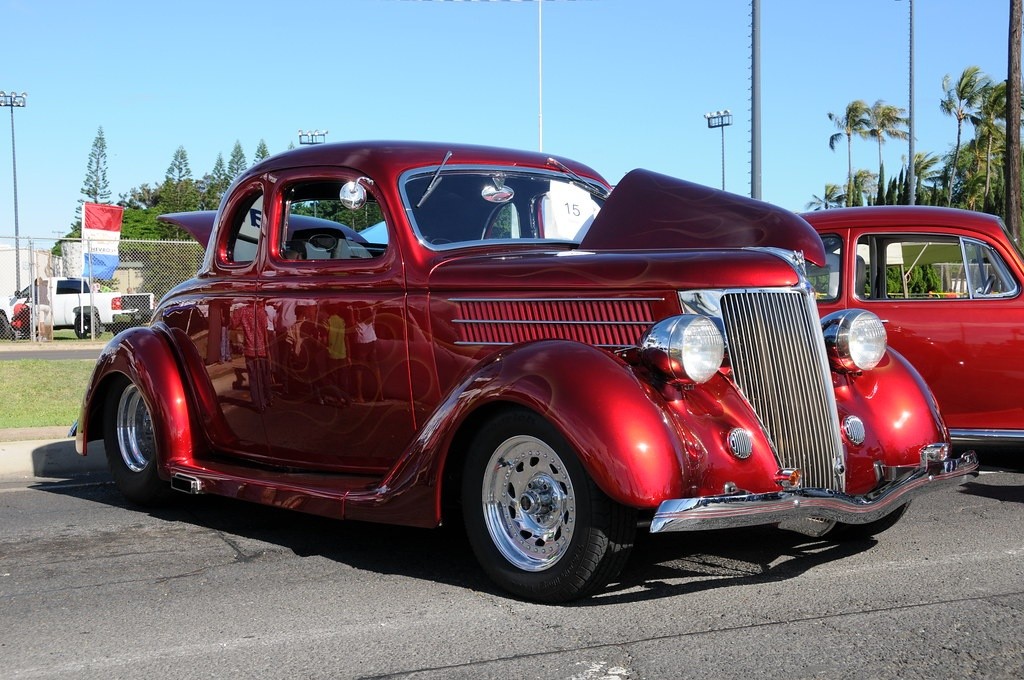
[7,273,155,339]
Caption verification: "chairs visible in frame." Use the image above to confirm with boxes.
[856,256,867,298]
[288,238,372,259]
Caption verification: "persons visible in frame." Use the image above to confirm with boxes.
[31,277,51,340]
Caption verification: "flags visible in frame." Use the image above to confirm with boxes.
[81,202,125,280]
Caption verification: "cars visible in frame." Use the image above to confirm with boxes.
[353,180,603,245]
[793,205,1024,449]
[67,140,982,607]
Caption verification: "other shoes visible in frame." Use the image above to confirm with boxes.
[376,396,384,402]
[226,356,232,362]
[351,397,366,403]
[218,360,225,365]
[265,398,275,407]
[318,398,325,406]
[250,403,265,413]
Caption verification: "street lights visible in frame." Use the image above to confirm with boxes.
[702,109,733,191]
[0,90,27,291]
[296,128,326,217]
